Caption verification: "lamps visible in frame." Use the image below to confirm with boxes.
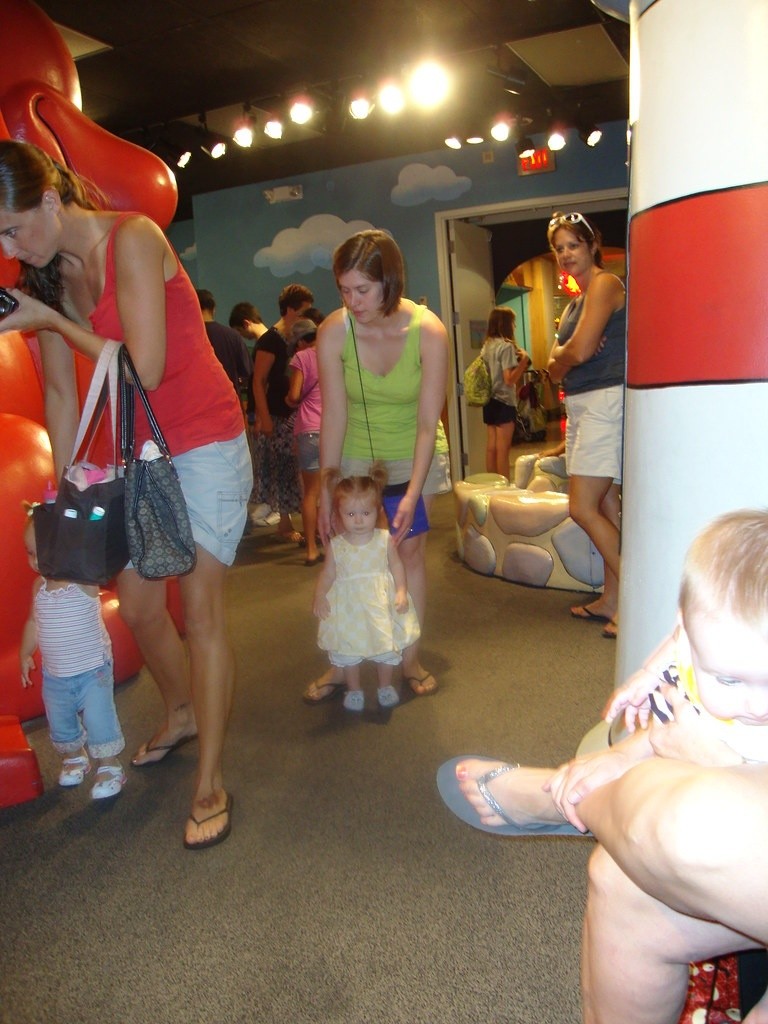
[169,54,603,168]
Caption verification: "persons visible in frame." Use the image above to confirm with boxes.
[194,289,248,429]
[0,138,254,850]
[482,307,530,482]
[604,507,768,761]
[436,685,768,1024]
[302,230,437,706]
[313,461,408,709]
[547,212,626,638]
[18,501,128,799]
[230,284,331,562]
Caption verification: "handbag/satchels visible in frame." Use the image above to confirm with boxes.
[32,340,124,585]
[117,344,196,581]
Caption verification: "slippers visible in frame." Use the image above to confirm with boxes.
[403,670,439,695]
[303,677,349,703]
[130,734,199,768]
[184,794,234,848]
[435,755,595,836]
[569,605,612,623]
[603,620,617,638]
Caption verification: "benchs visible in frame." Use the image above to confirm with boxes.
[455,472,603,593]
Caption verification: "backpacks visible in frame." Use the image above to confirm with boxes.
[465,340,510,407]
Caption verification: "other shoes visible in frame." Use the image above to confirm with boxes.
[298,531,323,547]
[256,510,291,526]
[377,686,400,706]
[276,530,304,543]
[92,757,128,798]
[251,503,272,520]
[58,755,92,786]
[343,691,365,711]
[305,552,324,565]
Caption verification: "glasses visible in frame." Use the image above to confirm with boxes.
[549,212,595,241]
